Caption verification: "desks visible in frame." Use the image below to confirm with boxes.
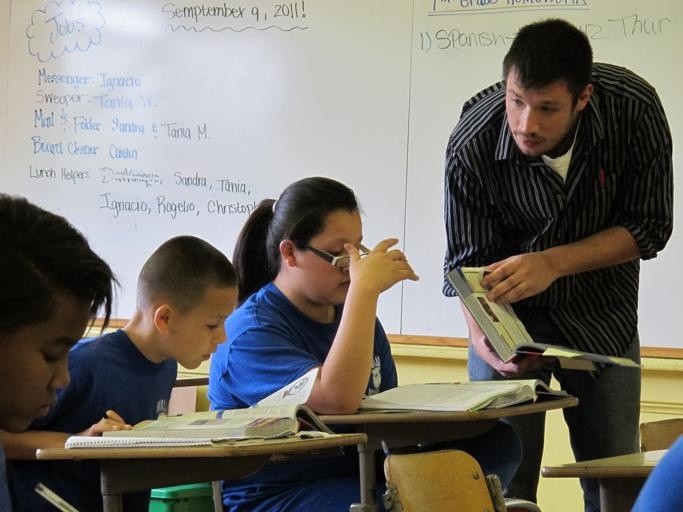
[317,396,580,512]
[540,448,669,512]
[36,432,368,512]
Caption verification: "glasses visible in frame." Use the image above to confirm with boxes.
[302,244,373,268]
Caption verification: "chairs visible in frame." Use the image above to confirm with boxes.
[381,451,508,511]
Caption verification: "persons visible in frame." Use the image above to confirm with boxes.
[437,18,674,512]
[215,174,420,512]
[631,436,683,512]
[1,194,121,512]
[1,235,240,512]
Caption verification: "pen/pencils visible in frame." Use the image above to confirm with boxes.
[24,477,81,512]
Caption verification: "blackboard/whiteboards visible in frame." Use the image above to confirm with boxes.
[0,0,683,359]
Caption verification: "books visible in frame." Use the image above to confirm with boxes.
[357,376,562,412]
[64,433,238,447]
[102,365,334,438]
[445,267,641,369]
